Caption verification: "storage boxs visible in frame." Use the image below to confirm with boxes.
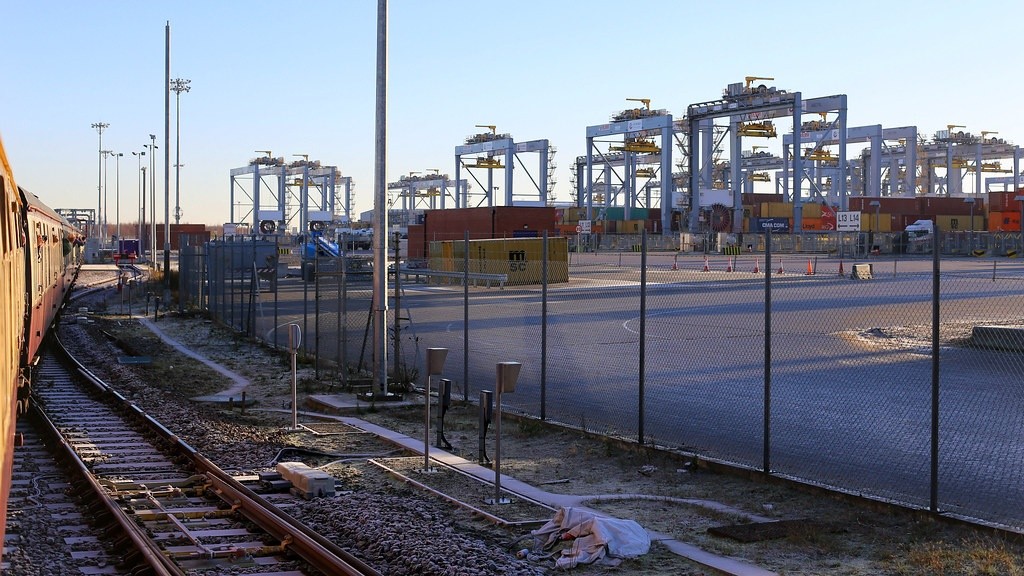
[137,188,1024,285]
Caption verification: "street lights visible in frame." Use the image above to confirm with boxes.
[111,153,124,238]
[1013,195,1024,237]
[963,198,975,231]
[870,202,880,233]
[133,151,146,257]
[90,122,111,237]
[144,144,158,267]
[150,133,158,273]
[99,149,114,242]
[168,77,192,221]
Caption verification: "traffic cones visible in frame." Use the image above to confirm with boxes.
[726,257,733,273]
[670,255,680,271]
[777,259,785,275]
[701,256,710,272]
[836,260,845,277]
[803,260,815,275]
[752,256,760,274]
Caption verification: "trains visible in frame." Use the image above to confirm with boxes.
[1,135,86,552]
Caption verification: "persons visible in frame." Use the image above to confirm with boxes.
[63,234,75,256]
[73,236,86,247]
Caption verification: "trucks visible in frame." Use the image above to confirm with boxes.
[908,220,989,255]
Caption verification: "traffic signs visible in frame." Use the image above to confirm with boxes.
[837,211,861,233]
